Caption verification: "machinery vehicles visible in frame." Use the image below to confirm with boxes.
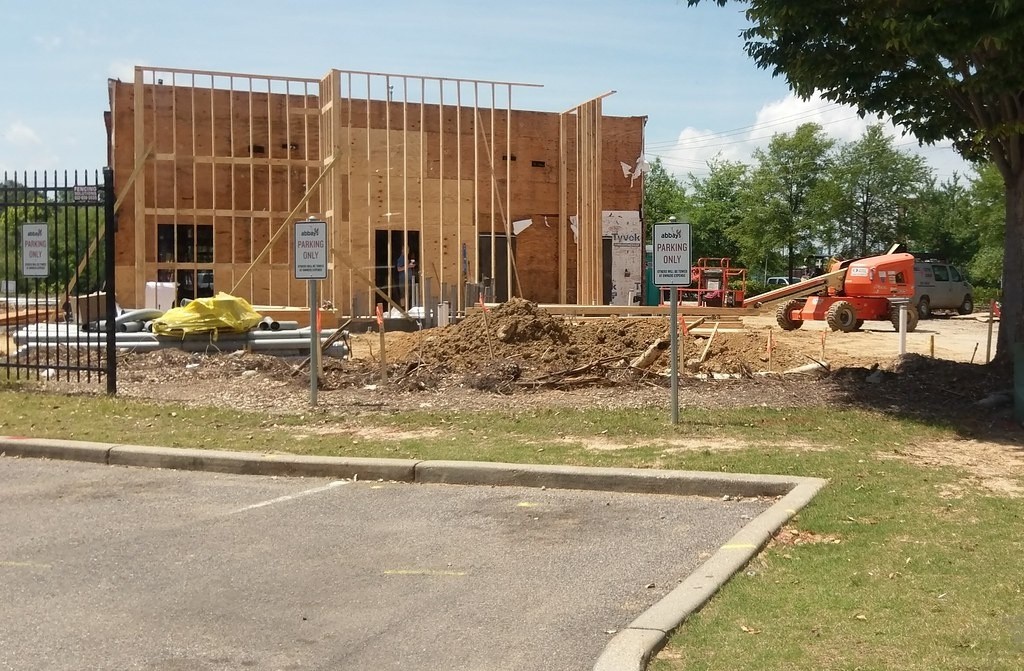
[659,243,919,333]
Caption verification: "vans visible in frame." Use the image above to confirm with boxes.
[912,263,974,319]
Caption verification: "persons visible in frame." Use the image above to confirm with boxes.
[397,245,415,310]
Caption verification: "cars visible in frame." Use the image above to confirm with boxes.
[767,277,801,285]
